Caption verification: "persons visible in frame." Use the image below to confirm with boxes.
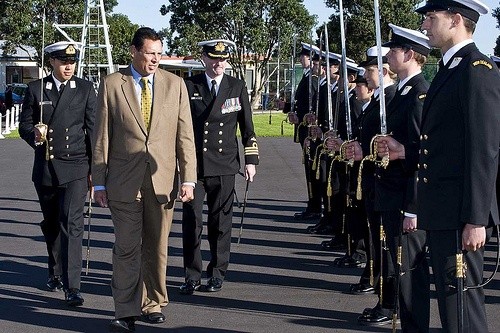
[415,0,500,333]
[326,47,402,325]
[5,86,13,110]
[277,42,376,294]
[177,40,259,295]
[91,27,197,333]
[18,42,98,305]
[343,23,432,333]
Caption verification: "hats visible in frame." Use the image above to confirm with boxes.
[382,23,434,57]
[415,0,489,23]
[298,41,369,84]
[198,40,235,59]
[44,42,82,61]
[357,46,391,67]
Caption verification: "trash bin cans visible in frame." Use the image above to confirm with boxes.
[263,94,273,110]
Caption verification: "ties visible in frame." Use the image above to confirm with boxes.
[141,77,153,133]
[210,80,216,102]
[60,84,66,95]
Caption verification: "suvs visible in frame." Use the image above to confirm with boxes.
[5,83,28,105]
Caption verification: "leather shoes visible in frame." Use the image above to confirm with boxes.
[65,288,84,307]
[206,276,223,291]
[295,212,321,220]
[357,307,400,327]
[46,275,63,292]
[177,279,201,293]
[333,254,367,268]
[141,312,166,324]
[343,283,376,295]
[321,233,347,252]
[308,221,335,236]
[112,317,135,333]
